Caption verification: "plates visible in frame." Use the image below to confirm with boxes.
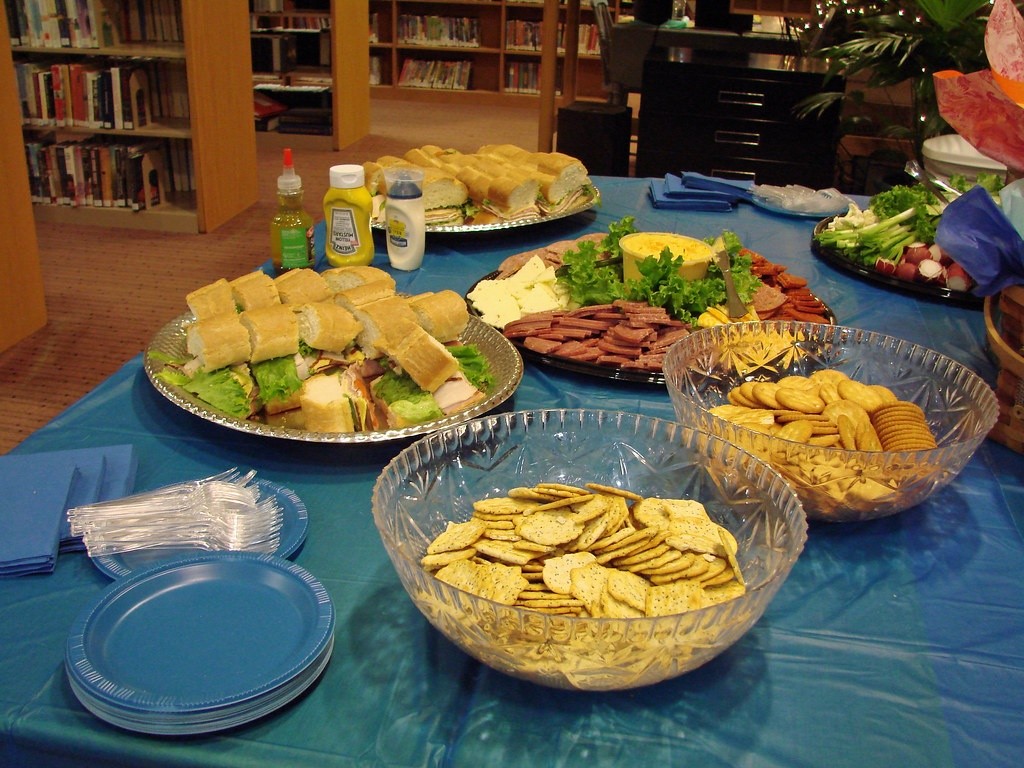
[750,196,849,218]
[372,184,603,232]
[85,474,311,582]
[140,307,525,444]
[63,550,339,736]
[809,213,985,314]
[462,263,839,384]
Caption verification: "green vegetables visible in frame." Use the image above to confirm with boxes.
[812,172,1004,267]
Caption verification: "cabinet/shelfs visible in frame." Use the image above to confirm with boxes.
[249,0,372,152]
[641,59,846,192]
[3,0,262,236]
[370,1,607,104]
[0,1,52,358]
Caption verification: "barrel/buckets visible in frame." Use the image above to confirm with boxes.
[980,285,1024,456]
[919,131,1008,185]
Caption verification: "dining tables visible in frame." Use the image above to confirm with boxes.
[0,175,1024,767]
[609,22,801,106]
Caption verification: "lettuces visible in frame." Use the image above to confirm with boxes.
[551,214,762,329]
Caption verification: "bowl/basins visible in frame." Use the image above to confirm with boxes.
[368,405,813,691]
[661,318,1002,522]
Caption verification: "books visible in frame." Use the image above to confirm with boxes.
[3,0,195,213]
[248,0,335,136]
[368,0,615,99]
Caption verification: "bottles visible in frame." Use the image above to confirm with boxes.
[323,164,375,266]
[269,148,316,272]
[382,167,426,270]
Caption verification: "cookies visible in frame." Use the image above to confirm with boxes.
[421,482,747,676]
[706,370,941,519]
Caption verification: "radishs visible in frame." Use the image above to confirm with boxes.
[873,241,973,291]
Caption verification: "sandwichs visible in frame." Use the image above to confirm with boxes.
[149,268,499,434]
[361,144,602,224]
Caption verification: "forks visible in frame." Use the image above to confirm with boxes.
[744,182,850,213]
[61,462,285,556]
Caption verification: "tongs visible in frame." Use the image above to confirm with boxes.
[903,159,964,207]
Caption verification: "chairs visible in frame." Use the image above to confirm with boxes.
[590,0,614,82]
